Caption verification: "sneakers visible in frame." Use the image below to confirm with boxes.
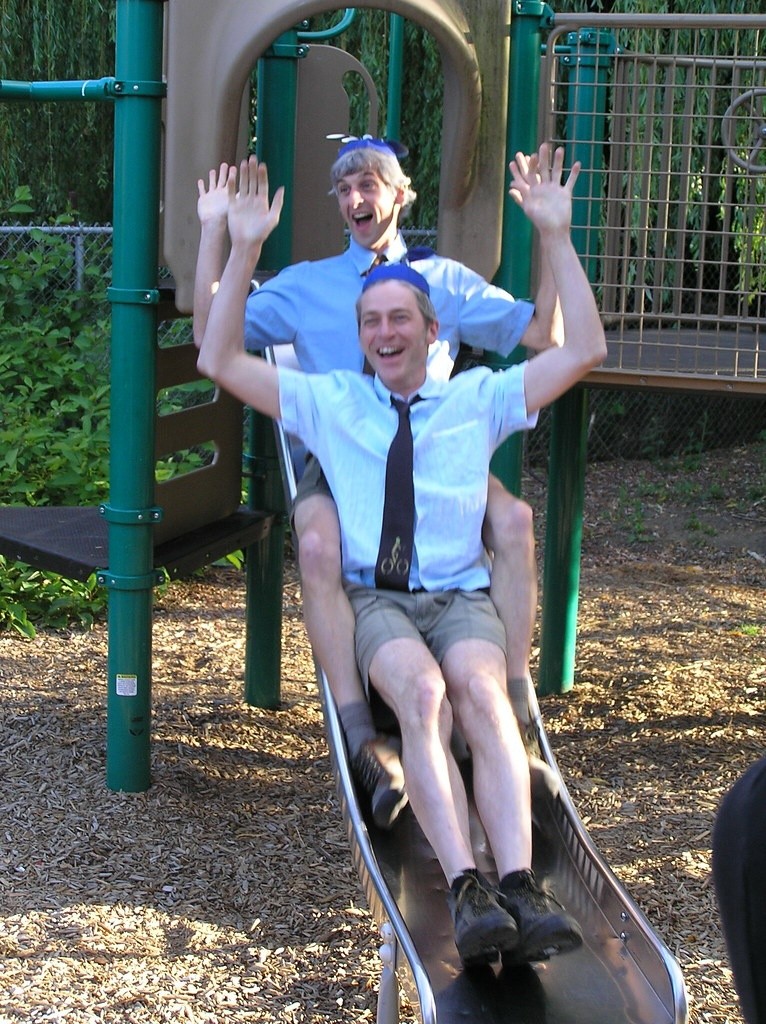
[520,720,560,801]
[352,732,409,828]
[499,868,583,962]
[447,868,519,966]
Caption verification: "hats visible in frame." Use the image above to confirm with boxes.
[363,263,430,296]
[338,139,397,159]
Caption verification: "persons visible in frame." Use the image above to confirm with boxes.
[197,141,608,968]
[193,140,564,862]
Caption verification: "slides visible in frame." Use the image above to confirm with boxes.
[243,268,696,1024]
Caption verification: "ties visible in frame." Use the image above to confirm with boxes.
[374,393,425,593]
[365,255,388,278]
